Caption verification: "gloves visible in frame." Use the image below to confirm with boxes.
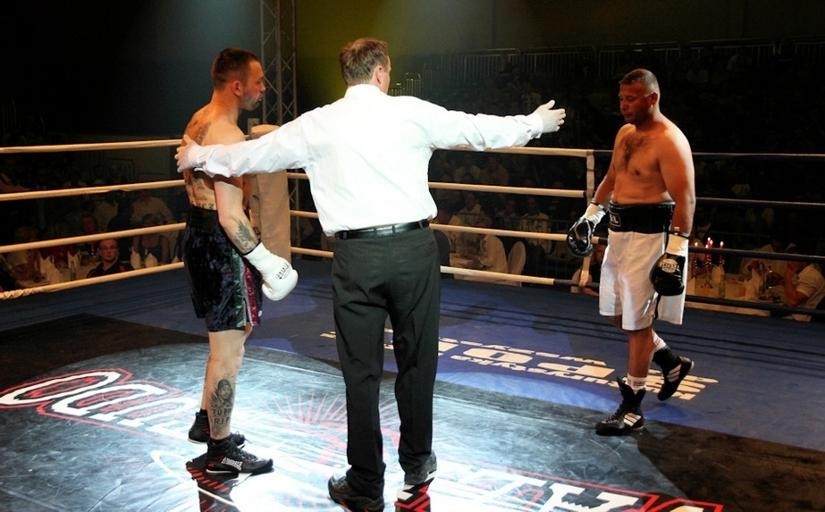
[649,231,685,297]
[567,202,606,255]
[244,240,300,302]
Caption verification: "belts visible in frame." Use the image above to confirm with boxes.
[335,219,430,240]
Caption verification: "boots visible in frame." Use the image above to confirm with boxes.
[596,377,647,435]
[652,347,694,402]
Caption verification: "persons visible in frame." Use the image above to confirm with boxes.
[566,68,698,437]
[428,149,607,294]
[174,36,567,512]
[176,46,302,476]
[0,165,175,293]
[772,243,825,322]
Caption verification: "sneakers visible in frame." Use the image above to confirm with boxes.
[206,438,273,474]
[402,449,438,486]
[187,411,246,449]
[328,464,384,512]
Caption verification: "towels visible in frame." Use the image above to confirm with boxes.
[13,244,163,289]
